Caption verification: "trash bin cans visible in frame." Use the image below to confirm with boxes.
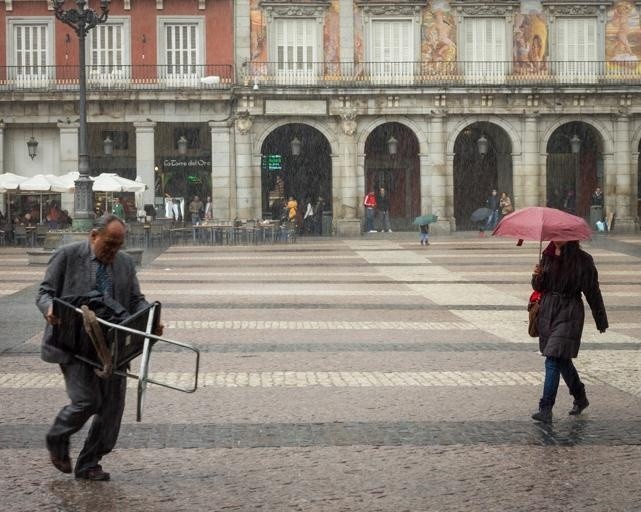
[321,211,333,236]
[589,205,603,230]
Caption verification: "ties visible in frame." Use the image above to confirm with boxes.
[95,261,109,293]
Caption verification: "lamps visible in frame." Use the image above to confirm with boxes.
[179,125,189,155]
[104,130,114,155]
[387,122,401,155]
[478,123,491,155]
[26,124,39,161]
[289,125,303,158]
[571,126,583,153]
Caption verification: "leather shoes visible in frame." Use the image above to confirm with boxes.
[80,464,110,481]
[46,438,73,474]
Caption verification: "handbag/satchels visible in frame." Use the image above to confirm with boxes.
[527,291,542,338]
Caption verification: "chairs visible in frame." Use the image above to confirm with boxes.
[44,292,202,422]
[0,211,294,258]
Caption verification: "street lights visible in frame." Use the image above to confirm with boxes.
[51,0,115,233]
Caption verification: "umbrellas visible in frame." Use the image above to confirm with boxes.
[492,206,592,263]
[470,208,493,223]
[0,172,147,223]
[411,215,438,226]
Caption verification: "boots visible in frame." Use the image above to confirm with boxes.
[532,406,554,424]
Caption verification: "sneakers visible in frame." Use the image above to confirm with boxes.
[568,397,590,415]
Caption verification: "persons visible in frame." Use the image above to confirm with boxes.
[189,195,204,225]
[546,186,603,215]
[377,187,392,233]
[364,190,378,233]
[486,189,499,229]
[0,197,126,229]
[204,196,213,218]
[532,241,607,421]
[611,5,632,55]
[272,196,325,234]
[420,224,430,245]
[499,192,512,217]
[36,213,164,480]
[478,219,486,239]
[425,12,451,69]
[515,9,547,73]
